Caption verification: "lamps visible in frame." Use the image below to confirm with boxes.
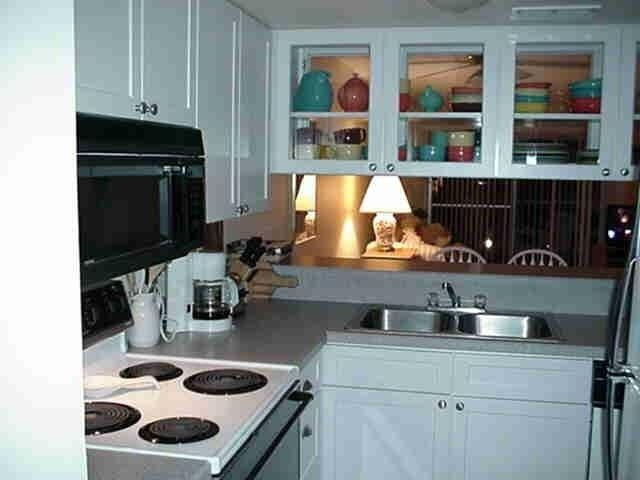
[358,175,413,252]
[295,174,316,237]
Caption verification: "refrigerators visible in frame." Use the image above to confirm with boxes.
[604,183,640,480]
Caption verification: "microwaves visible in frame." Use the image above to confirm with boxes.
[81,110,207,287]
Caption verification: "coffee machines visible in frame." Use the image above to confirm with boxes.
[167,252,233,335]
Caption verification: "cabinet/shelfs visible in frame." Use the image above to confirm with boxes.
[317,342,593,479]
[74,1,268,224]
[265,24,640,182]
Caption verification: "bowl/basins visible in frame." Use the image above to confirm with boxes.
[295,126,323,158]
[419,130,481,161]
[269,241,294,255]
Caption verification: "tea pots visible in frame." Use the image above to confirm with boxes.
[291,68,331,111]
[126,293,163,347]
[336,71,369,112]
[417,83,446,114]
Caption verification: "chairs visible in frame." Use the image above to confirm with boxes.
[432,246,489,264]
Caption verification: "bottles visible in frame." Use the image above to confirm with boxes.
[586,118,600,151]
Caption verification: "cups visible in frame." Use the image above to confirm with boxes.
[334,146,362,160]
[334,127,367,144]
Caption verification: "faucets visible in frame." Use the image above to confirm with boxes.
[441,281,461,307]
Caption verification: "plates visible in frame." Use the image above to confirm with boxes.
[448,85,480,112]
[514,140,571,165]
[515,77,600,112]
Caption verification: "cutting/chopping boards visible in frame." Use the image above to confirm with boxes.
[229,257,299,303]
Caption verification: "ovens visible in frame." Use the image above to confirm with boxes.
[213,374,315,480]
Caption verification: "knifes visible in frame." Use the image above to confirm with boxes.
[239,237,265,266]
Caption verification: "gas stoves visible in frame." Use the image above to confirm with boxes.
[84,332,301,475]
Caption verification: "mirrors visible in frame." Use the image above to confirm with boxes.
[290,175,319,246]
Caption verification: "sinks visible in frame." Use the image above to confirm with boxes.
[454,309,570,345]
[342,303,454,336]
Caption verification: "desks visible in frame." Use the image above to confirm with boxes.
[360,238,418,262]
